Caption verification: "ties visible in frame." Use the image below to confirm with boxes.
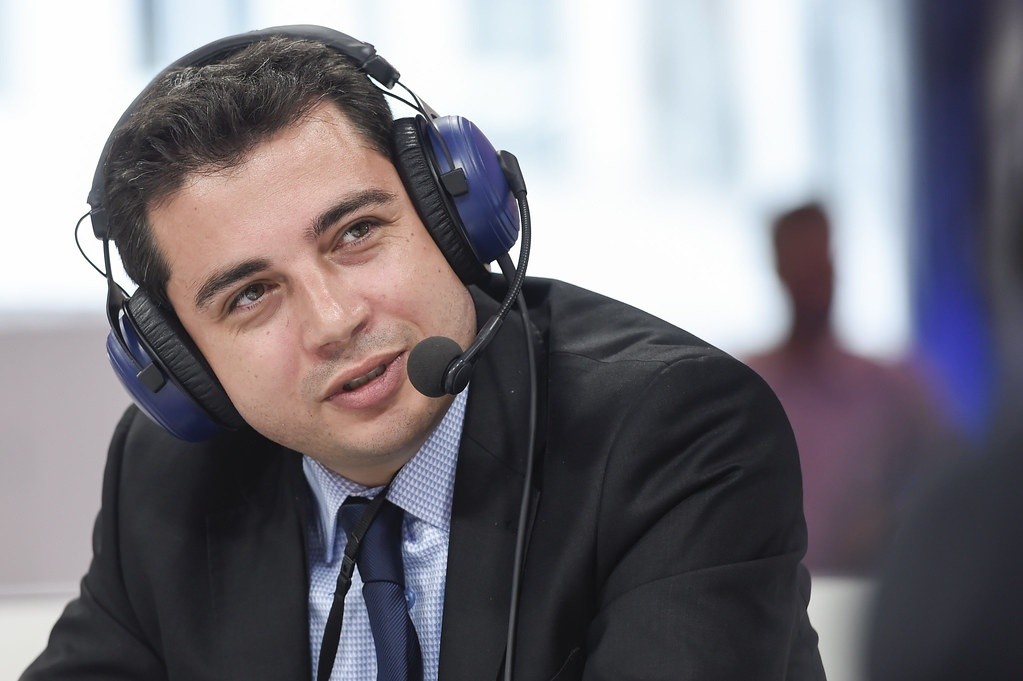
[338,494,424,681]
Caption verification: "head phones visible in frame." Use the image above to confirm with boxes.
[75,25,528,443]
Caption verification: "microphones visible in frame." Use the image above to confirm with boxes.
[407,197,531,399]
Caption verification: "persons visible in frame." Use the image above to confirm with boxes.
[22,25,829,681]
[730,199,954,577]
